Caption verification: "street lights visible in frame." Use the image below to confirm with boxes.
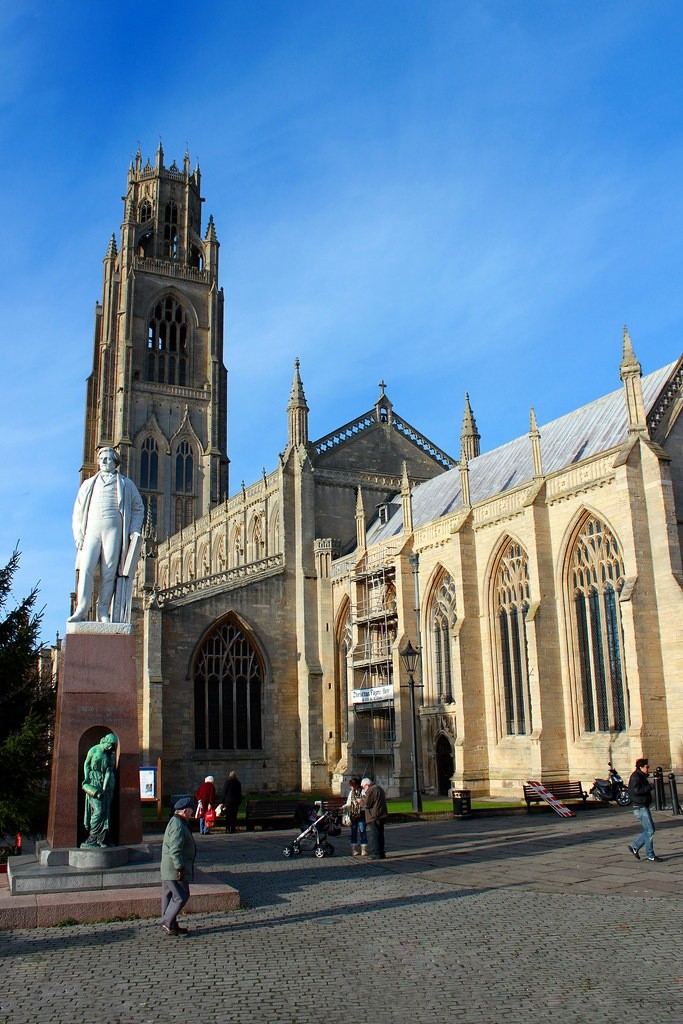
[398,638,423,814]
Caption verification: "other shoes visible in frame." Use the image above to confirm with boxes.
[628,845,640,860]
[646,856,663,862]
[367,853,385,860]
[226,828,235,834]
[160,922,187,935]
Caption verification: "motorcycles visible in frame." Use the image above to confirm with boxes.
[590,762,631,807]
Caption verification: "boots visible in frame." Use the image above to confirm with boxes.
[361,844,368,856]
[351,843,359,856]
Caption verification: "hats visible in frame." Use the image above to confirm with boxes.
[174,797,194,810]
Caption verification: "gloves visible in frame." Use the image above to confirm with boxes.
[650,783,655,790]
[177,869,185,880]
[208,804,211,810]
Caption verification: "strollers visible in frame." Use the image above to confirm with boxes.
[283,799,342,858]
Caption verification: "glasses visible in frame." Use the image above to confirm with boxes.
[646,766,649,769]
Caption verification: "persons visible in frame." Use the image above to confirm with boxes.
[309,809,317,821]
[194,775,216,834]
[82,733,118,847]
[357,778,389,859]
[628,759,663,862]
[220,771,242,834]
[338,778,368,856]
[68,447,145,623]
[158,798,197,936]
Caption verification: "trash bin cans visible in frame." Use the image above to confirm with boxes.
[451,790,473,819]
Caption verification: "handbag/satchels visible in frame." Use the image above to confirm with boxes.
[342,804,352,827]
[205,809,216,828]
[194,799,206,819]
[328,817,341,835]
